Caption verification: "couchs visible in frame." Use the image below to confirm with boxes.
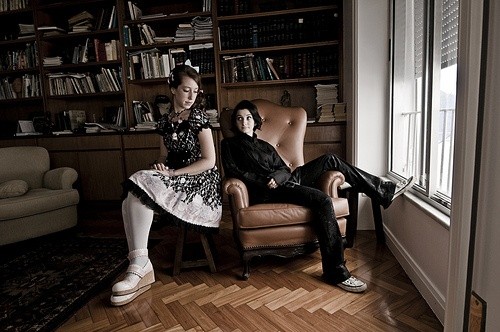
[220,99,350,280]
[0,146,80,246]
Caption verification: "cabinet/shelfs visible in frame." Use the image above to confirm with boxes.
[211,0,352,206]
[0,0,46,150]
[32,0,126,201]
[117,0,222,181]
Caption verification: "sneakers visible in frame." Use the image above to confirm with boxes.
[391,176,414,202]
[337,275,367,292]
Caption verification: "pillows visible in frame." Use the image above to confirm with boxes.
[0,180,29,198]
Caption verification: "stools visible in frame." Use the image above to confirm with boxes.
[172,226,217,275]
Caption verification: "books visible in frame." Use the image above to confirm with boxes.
[217,0,343,84]
[204,109,220,128]
[129,99,159,132]
[0,0,214,100]
[51,107,124,134]
[314,84,346,123]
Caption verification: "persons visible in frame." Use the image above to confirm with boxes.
[221,100,415,292]
[110,65,222,306]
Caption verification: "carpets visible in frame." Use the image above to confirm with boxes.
[0,236,163,332]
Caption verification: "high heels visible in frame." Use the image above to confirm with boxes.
[111,284,151,306]
[112,249,155,295]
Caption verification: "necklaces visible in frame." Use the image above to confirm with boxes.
[168,109,186,142]
